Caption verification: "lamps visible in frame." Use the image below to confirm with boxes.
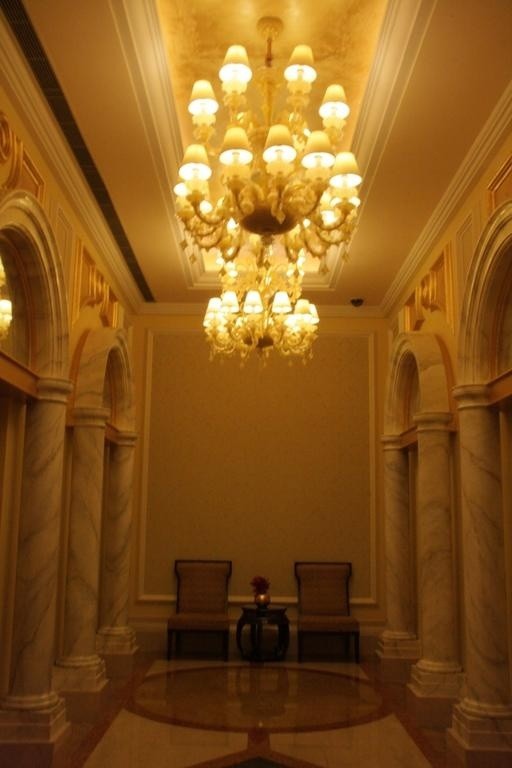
[168,14,369,368]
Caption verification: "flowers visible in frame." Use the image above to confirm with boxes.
[251,574,271,590]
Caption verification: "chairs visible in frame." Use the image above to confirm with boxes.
[170,558,237,658]
[294,558,363,664]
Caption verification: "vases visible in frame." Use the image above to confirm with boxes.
[255,589,270,607]
